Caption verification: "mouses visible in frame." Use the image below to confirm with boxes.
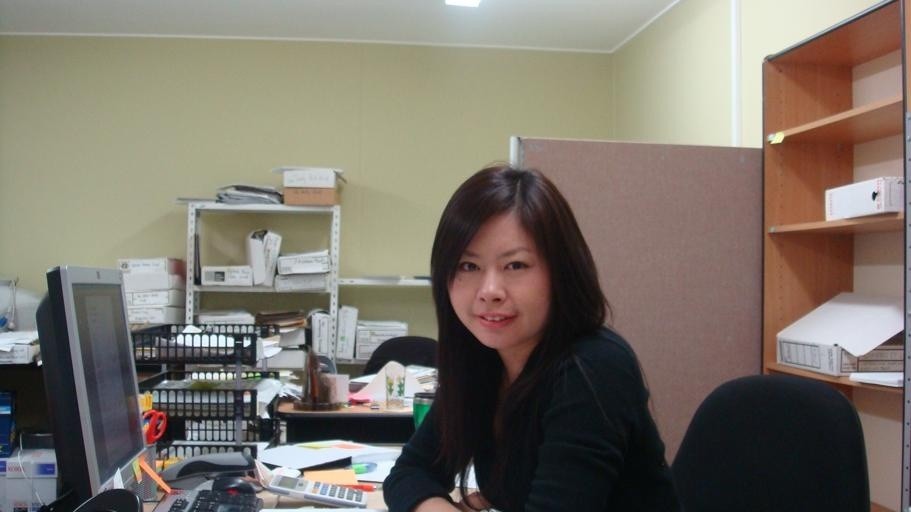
[212,476,254,496]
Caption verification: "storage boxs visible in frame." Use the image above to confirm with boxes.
[1,444,76,511]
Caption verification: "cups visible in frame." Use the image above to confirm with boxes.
[385,366,406,412]
[413,393,435,430]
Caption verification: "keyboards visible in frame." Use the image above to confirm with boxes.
[152,490,262,512]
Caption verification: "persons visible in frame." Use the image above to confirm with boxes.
[381,161,687,512]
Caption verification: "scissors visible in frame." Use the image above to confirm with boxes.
[143,409,168,444]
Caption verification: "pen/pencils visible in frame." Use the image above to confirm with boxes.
[339,484,377,492]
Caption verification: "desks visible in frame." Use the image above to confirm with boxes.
[142,441,402,512]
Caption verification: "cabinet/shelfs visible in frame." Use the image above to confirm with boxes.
[757,2,911,510]
[185,204,341,374]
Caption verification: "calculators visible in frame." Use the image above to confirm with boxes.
[268,474,367,509]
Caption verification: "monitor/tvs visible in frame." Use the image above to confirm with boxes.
[34,265,149,498]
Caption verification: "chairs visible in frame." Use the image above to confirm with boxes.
[670,376,871,511]
